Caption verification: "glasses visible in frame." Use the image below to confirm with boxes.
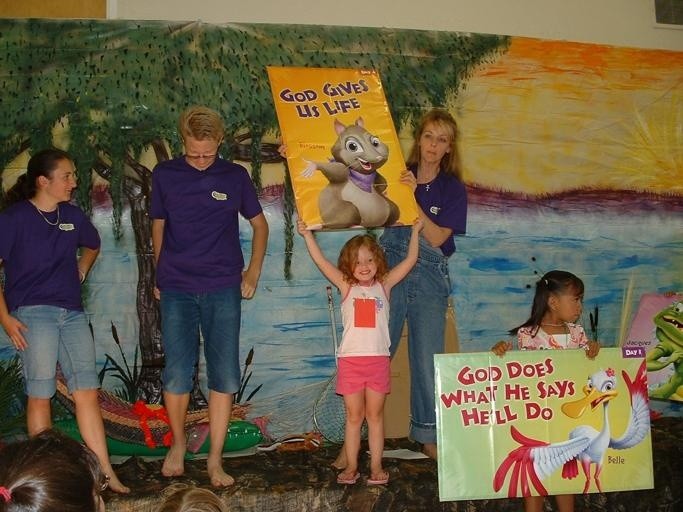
[184,152,218,160]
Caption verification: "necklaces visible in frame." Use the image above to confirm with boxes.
[420,165,436,183]
[32,196,60,225]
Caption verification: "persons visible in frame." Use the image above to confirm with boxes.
[277,108,467,470]
[151,106,269,485]
[492,271,601,511]
[0,428,110,512]
[0,148,130,494]
[296,215,424,483]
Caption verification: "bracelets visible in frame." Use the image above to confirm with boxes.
[79,269,85,284]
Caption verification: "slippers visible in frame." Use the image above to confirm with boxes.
[336,470,363,485]
[366,470,390,485]
